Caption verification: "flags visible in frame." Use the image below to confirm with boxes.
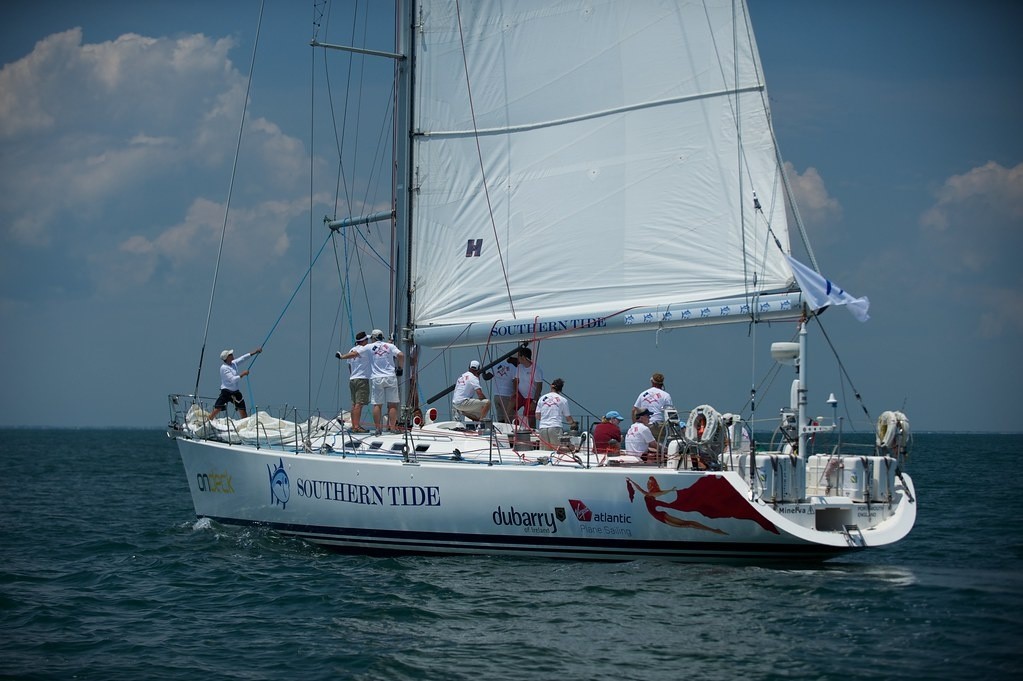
[784,255,871,323]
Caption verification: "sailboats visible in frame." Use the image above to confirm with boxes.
[164,2,920,562]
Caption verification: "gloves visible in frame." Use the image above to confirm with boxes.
[396,366,403,376]
[335,351,342,359]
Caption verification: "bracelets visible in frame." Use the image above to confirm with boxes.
[533,400,537,404]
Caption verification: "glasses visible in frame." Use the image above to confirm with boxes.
[518,353,522,357]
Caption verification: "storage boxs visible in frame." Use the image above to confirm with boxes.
[717,450,898,502]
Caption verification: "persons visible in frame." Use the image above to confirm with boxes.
[482,357,521,424]
[453,360,491,432]
[504,348,543,429]
[535,378,576,451]
[592,411,624,454]
[722,412,751,452]
[632,373,673,442]
[625,408,668,462]
[208,348,263,420]
[336,329,404,436]
[347,332,394,432]
[679,421,686,434]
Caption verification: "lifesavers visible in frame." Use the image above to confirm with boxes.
[876,409,911,447]
[685,403,725,443]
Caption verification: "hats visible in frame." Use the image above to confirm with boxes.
[370,329,383,338]
[605,410,624,421]
[635,409,654,419]
[469,360,480,371]
[509,352,518,358]
[220,349,233,360]
[355,333,372,341]
[722,413,733,425]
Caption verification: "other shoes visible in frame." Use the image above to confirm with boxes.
[451,407,462,421]
[352,425,370,433]
[476,422,491,432]
